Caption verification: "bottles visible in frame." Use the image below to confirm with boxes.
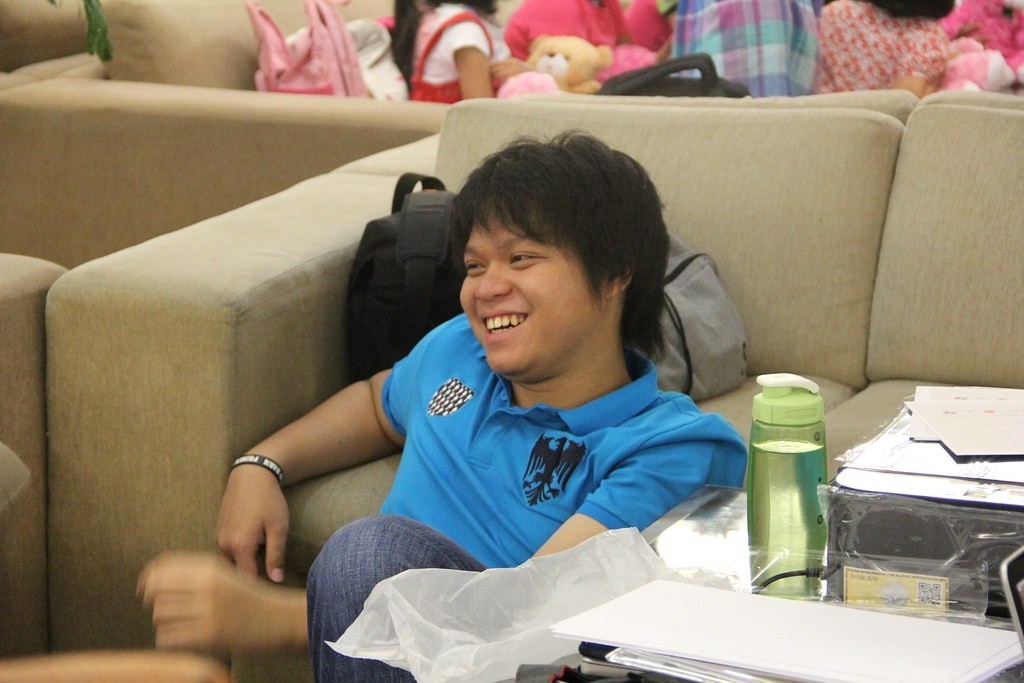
[749,373,828,600]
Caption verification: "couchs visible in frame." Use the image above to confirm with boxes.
[0,0,1022,682]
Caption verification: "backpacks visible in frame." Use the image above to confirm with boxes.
[344,171,466,383]
[640,229,747,400]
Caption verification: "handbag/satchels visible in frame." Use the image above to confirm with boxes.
[590,53,749,98]
[408,12,499,104]
[244,0,370,97]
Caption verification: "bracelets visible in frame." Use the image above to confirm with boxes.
[230,454,284,484]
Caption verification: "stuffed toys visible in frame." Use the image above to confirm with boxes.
[528,36,612,96]
[938,37,1016,93]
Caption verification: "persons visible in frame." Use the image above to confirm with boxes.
[507,0,1023,97]
[134,129,751,683]
[394,0,531,103]
